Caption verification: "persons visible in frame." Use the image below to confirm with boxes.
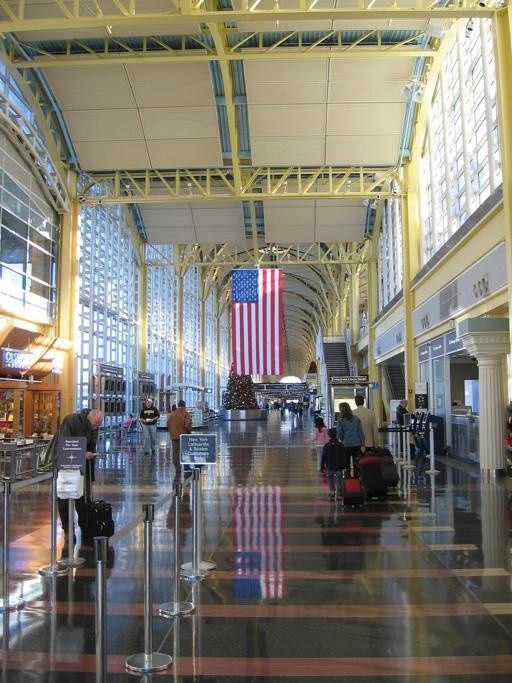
[320,428,347,501]
[334,401,366,479]
[351,394,381,449]
[41,407,105,555]
[166,399,193,473]
[121,413,134,430]
[279,398,304,418]
[138,397,161,455]
[302,416,332,472]
[395,397,416,457]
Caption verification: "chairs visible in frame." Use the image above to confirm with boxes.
[330,493,342,501]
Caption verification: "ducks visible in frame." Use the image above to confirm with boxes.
[364,447,400,488]
[358,455,386,498]
[341,478,364,505]
[86,498,115,539]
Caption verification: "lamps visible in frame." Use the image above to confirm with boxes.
[114,419,143,438]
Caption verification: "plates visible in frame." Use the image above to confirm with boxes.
[31,384,58,437]
[14,385,34,437]
[1,380,19,439]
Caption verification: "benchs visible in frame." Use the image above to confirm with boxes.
[228,480,287,602]
[230,265,285,377]
